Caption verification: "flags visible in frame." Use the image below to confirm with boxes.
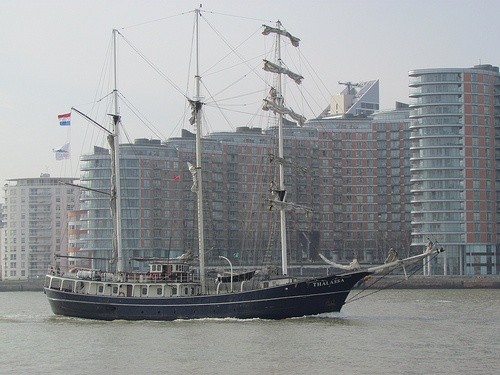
[58,113,72,126]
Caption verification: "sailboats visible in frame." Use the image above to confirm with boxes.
[40,3,446,322]
